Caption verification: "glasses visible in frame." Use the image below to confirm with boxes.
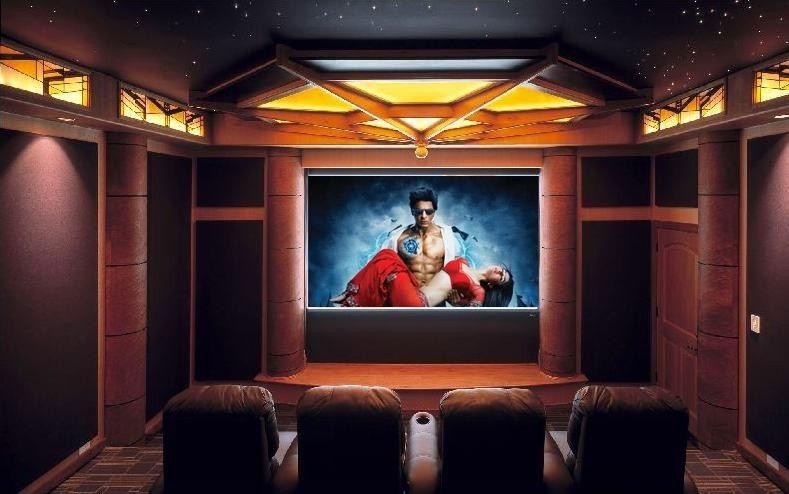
[413,208,434,216]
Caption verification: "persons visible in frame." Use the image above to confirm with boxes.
[378,187,466,307]
[329,248,514,307]
